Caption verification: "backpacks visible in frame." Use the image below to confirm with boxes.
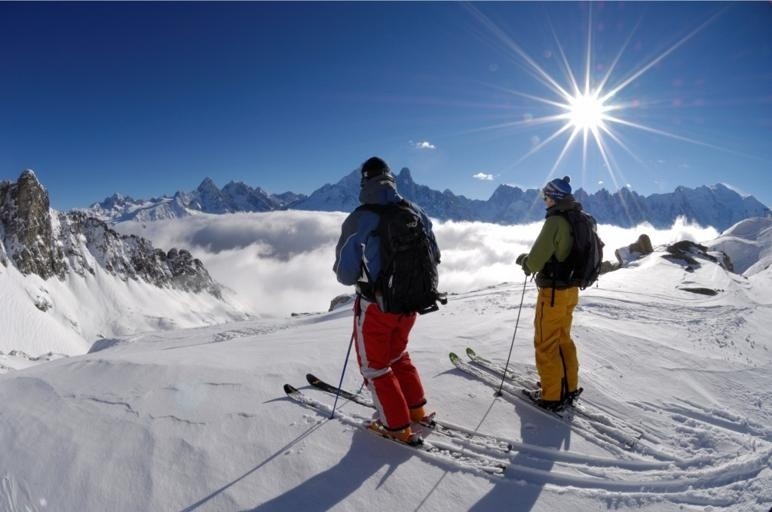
[545,212,603,289]
[356,200,441,313]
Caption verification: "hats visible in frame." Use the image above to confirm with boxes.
[361,156,396,188]
[545,176,573,198]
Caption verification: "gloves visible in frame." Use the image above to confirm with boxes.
[516,253,528,264]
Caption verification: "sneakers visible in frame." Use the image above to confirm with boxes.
[530,387,581,408]
[408,406,425,420]
[371,419,409,440]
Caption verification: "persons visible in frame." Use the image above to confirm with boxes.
[516,176,596,410]
[334,156,436,441]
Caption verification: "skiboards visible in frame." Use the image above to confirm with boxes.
[448,347,643,449]
[283,374,512,474]
